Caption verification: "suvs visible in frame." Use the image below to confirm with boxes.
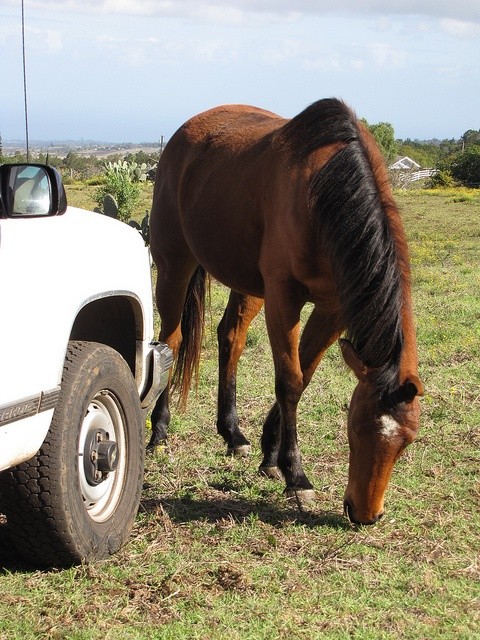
[0,163,175,572]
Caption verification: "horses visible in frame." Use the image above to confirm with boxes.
[146,97,427,528]
